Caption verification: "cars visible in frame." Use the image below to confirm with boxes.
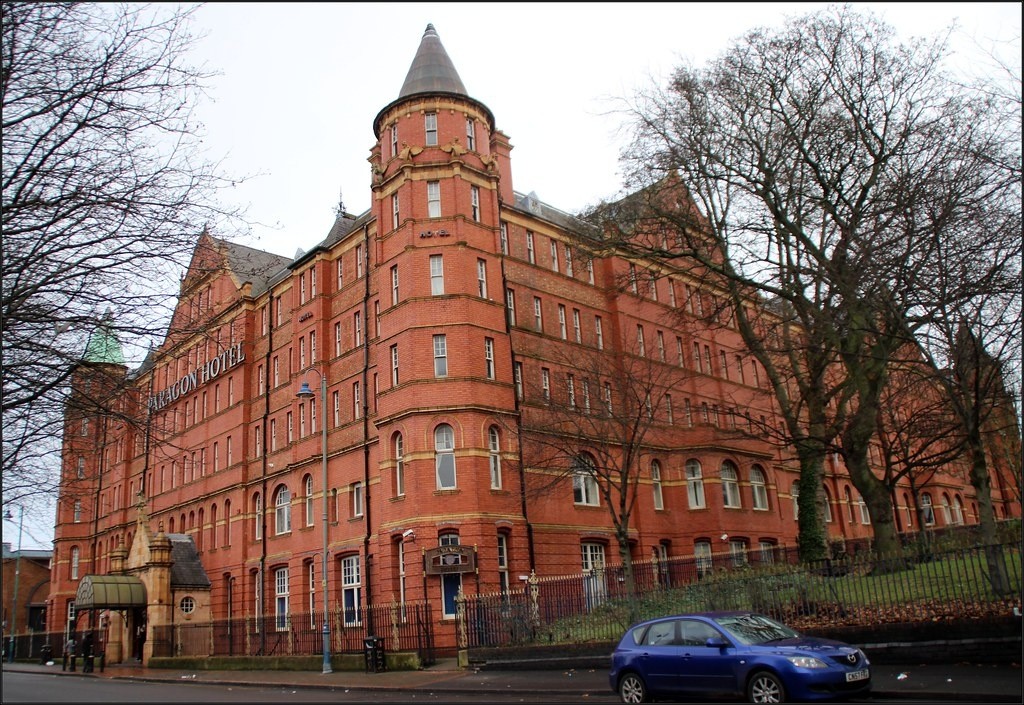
[608,612,873,703]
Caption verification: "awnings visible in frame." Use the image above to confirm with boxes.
[71,574,147,673]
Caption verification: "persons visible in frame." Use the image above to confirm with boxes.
[65,637,78,666]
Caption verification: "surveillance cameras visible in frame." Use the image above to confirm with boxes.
[402,530,414,537]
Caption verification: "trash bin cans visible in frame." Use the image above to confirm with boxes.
[40,645,51,664]
[364,636,387,673]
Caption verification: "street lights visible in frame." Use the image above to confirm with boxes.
[2,502,23,664]
[296,367,335,674]
